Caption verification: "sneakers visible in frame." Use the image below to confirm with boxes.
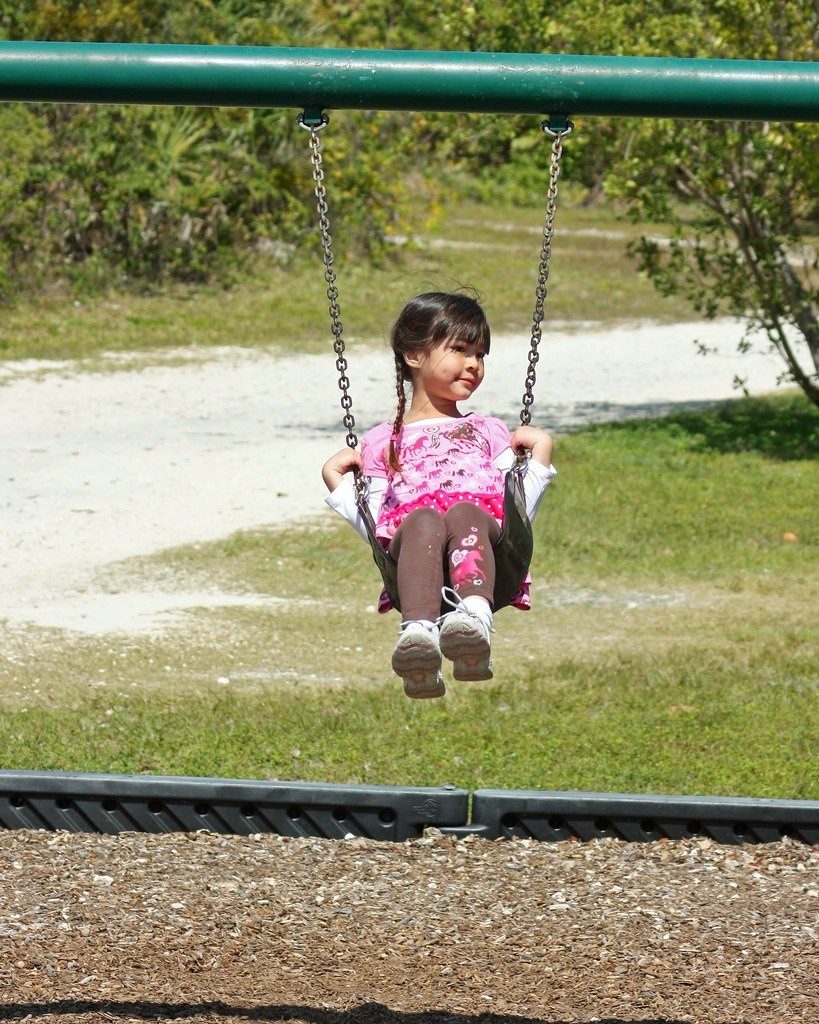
[392,621,445,699]
[435,587,496,683]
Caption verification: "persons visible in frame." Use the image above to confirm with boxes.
[321,291,557,699]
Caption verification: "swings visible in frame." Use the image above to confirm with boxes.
[293,110,575,620]
